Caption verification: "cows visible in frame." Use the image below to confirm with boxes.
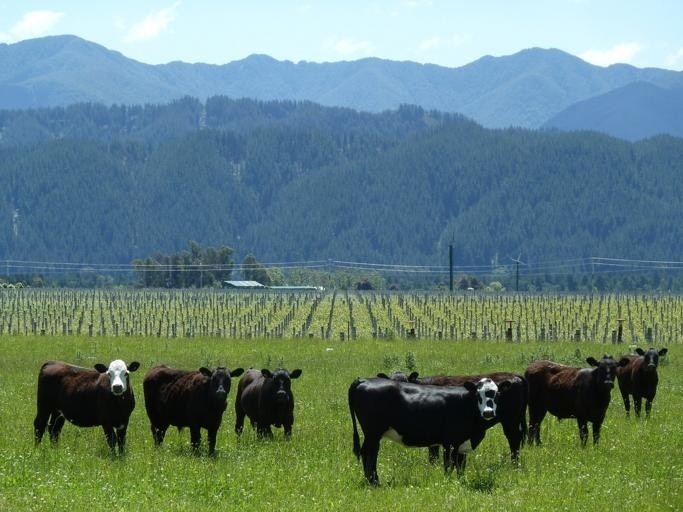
[234,366,302,445]
[524,353,631,451]
[616,347,668,420]
[33,359,140,462]
[348,376,512,488]
[376,371,529,480]
[143,363,245,459]
